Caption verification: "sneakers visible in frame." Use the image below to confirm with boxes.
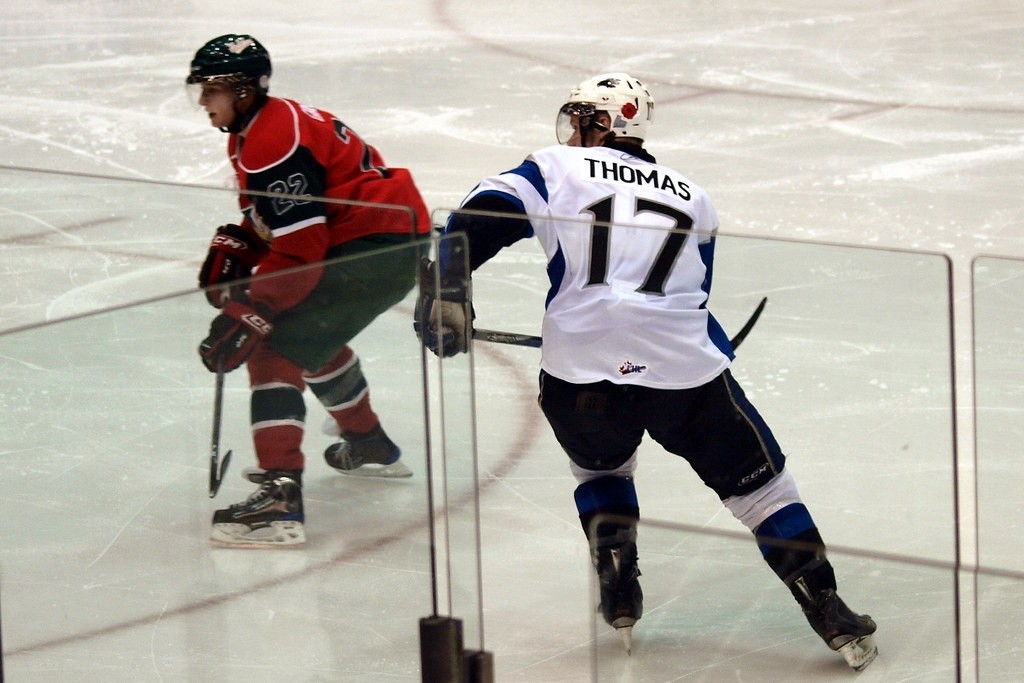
[211,469,305,545]
[591,540,641,655]
[325,426,413,478]
[786,561,879,670]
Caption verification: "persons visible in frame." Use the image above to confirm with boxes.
[184,35,431,550]
[413,73,879,672]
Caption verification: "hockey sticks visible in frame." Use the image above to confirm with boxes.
[434,296,767,355]
[207,281,236,501]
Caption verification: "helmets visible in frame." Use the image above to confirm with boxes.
[186,34,271,108]
[564,72,656,141]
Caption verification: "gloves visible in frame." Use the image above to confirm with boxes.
[199,292,271,374]
[198,224,254,308]
[413,289,475,356]
